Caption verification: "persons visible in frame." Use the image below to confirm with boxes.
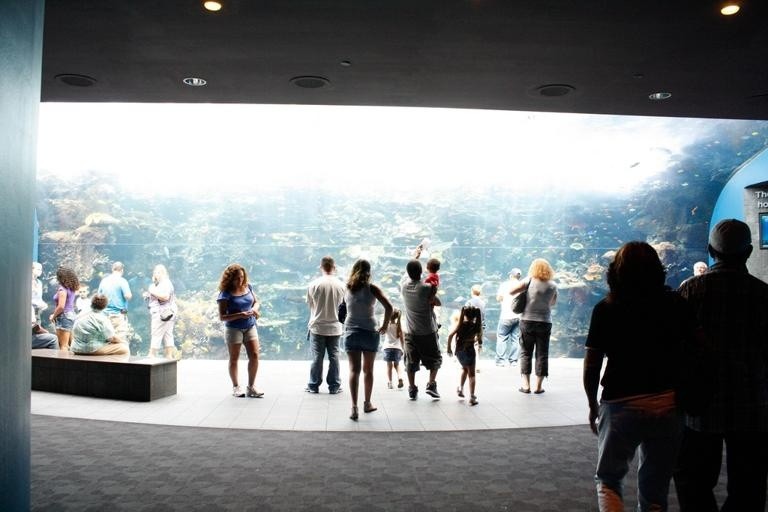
[48,267,79,350]
[380,307,406,389]
[303,255,345,394]
[69,293,131,357]
[399,242,443,400]
[581,240,683,512]
[421,257,442,331]
[32,276,60,350]
[678,260,709,289]
[216,263,265,398]
[463,283,485,373]
[341,257,393,420]
[141,263,178,359]
[672,217,767,511]
[495,267,522,366]
[31,261,48,327]
[75,283,90,313]
[95,261,133,341]
[446,304,484,405]
[508,257,558,394]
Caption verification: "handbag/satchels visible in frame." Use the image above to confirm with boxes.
[512,291,526,313]
[160,305,173,320]
[338,302,347,324]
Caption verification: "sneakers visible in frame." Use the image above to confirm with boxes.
[304,387,316,392]
[469,399,478,404]
[350,407,358,418]
[336,388,343,393]
[457,391,464,397]
[425,382,440,398]
[409,386,417,398]
[246,386,264,397]
[364,402,377,412]
[233,386,245,397]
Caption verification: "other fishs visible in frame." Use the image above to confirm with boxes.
[38,127,768,364]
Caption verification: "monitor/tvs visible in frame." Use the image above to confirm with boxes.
[759,212,768,250]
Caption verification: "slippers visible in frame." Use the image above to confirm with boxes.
[519,387,530,393]
[534,389,544,393]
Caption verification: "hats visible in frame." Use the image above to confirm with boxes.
[710,220,752,253]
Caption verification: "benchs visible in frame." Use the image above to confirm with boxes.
[31,346,184,403]
